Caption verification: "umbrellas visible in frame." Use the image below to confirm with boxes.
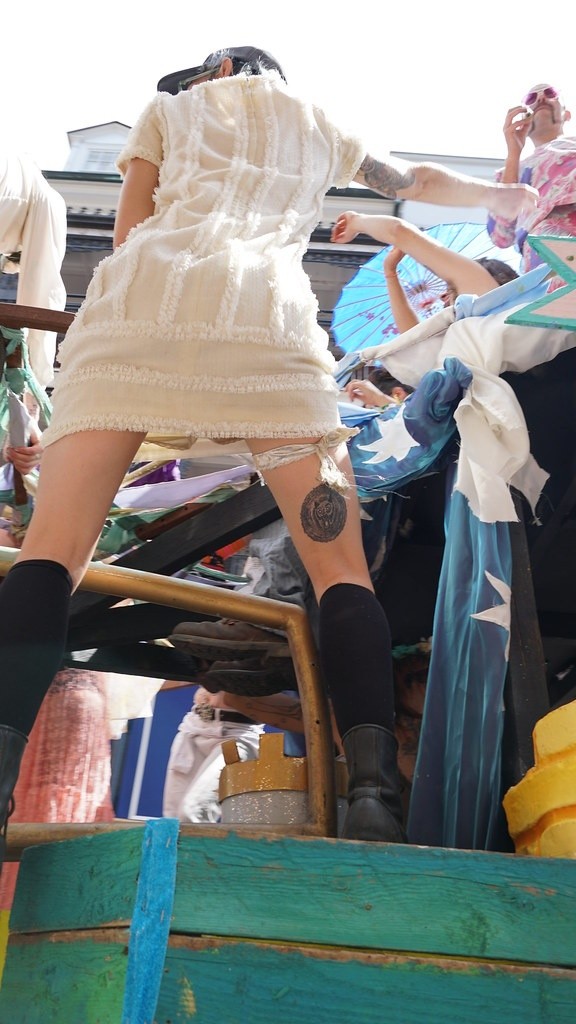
[329,221,523,368]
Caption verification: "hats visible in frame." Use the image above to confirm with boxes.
[157,46,288,95]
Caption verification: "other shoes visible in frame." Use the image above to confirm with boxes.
[205,661,297,696]
[169,616,292,667]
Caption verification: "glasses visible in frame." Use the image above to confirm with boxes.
[525,87,558,106]
[178,67,220,90]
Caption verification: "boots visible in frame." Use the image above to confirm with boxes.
[342,723,407,843]
[0,724,30,858]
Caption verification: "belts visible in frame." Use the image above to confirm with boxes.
[212,710,261,724]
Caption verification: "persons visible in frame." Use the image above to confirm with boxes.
[0,155,67,474]
[329,211,518,335]
[0,669,122,909]
[486,83,576,294]
[160,685,266,830]
[346,379,395,408]
[0,47,538,842]
